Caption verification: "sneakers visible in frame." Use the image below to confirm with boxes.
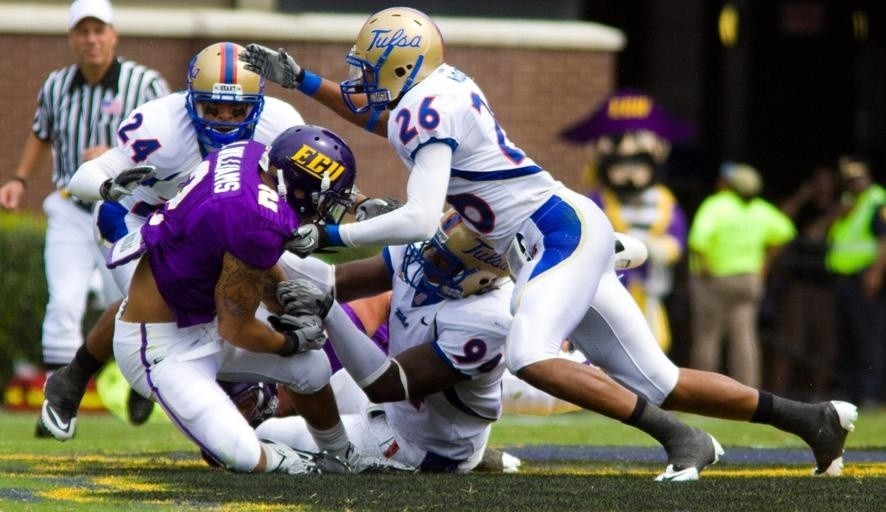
[653,431,726,485]
[34,416,54,441]
[470,445,523,474]
[343,442,417,475]
[127,387,155,426]
[810,399,859,477]
[43,368,78,442]
[260,438,351,477]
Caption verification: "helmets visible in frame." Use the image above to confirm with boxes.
[345,6,448,108]
[184,42,266,112]
[257,124,356,227]
[418,206,512,301]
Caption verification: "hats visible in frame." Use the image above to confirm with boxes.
[66,0,115,34]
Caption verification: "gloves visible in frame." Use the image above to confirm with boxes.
[276,278,334,318]
[238,43,305,92]
[267,312,328,357]
[282,223,327,259]
[356,196,402,222]
[98,165,158,202]
